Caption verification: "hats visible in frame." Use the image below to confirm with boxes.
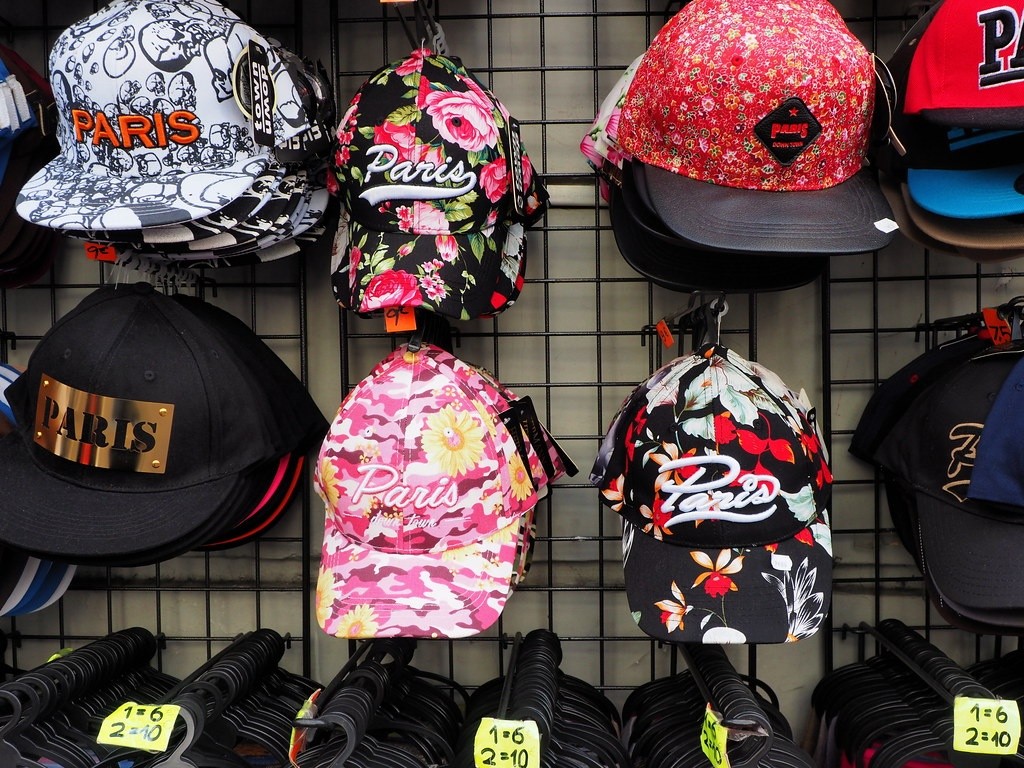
[0,288,327,570]
[581,1,899,298]
[590,345,835,646]
[848,298,1024,637]
[872,1,1024,259]
[310,345,568,640]
[16,0,337,270]
[329,45,552,327]
